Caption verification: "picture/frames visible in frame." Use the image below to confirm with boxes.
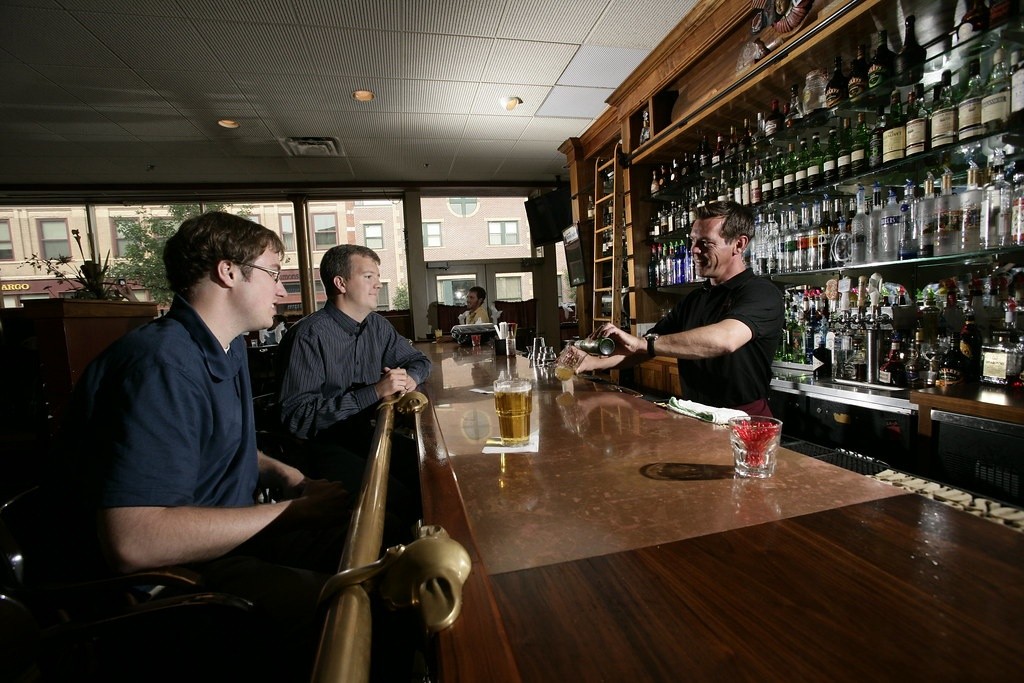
[561,222,587,288]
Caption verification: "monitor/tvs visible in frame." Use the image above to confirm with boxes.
[525,185,572,248]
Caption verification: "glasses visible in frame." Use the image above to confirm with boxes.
[233,260,282,280]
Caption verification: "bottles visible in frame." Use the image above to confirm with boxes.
[710,99,784,208]
[743,207,797,275]
[784,85,822,195]
[602,230,627,258]
[833,262,1024,388]
[640,111,710,288]
[574,338,615,356]
[587,196,595,218]
[866,173,934,263]
[931,0,1024,149]
[934,149,1024,257]
[834,189,866,267]
[506,324,516,356]
[797,194,834,272]
[774,289,828,364]
[602,201,613,227]
[869,15,931,172]
[823,45,869,184]
[603,170,614,197]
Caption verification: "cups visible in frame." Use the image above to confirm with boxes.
[495,339,506,355]
[494,378,534,447]
[435,330,442,340]
[555,340,588,381]
[471,335,481,347]
[537,347,545,366]
[729,415,783,478]
[533,337,545,367]
[543,346,556,366]
[251,339,258,348]
[555,392,591,435]
[526,346,533,360]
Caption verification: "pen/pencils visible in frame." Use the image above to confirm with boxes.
[494,322,518,340]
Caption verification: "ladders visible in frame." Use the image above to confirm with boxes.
[592,144,622,338]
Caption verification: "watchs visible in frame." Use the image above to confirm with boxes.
[645,332,660,357]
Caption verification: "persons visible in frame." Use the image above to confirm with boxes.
[436,286,489,344]
[280,243,430,439]
[68,210,347,572]
[555,200,783,431]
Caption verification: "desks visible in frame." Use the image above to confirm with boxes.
[248,343,279,355]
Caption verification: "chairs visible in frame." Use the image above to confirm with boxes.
[1,393,433,683]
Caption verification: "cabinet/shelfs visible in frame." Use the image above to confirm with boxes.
[554,1,1024,510]
